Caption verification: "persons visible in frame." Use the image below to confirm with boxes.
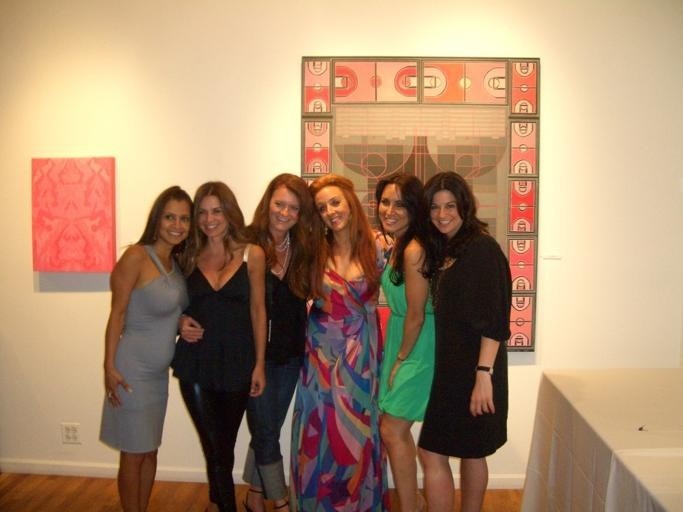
[290,173,395,512]
[377,175,436,512]
[241,174,314,512]
[169,182,266,512]
[414,172,512,512]
[99,186,191,512]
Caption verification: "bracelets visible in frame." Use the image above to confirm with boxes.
[475,366,494,375]
[398,352,405,361]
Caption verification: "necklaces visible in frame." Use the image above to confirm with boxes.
[267,231,290,275]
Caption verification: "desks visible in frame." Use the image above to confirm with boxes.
[516,365,681,512]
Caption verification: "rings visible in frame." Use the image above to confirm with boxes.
[108,393,111,398]
